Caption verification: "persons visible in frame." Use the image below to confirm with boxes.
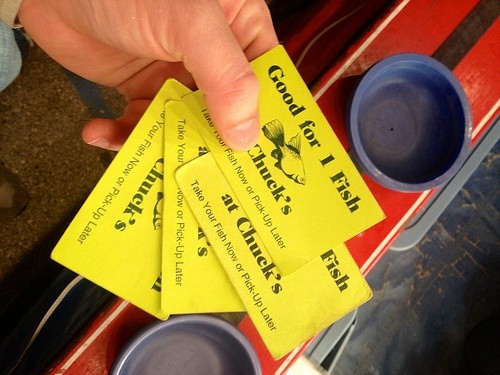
[1,0,279,151]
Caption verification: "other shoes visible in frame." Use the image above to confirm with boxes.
[0,160,29,219]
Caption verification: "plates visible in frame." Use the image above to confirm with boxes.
[111,315,265,374]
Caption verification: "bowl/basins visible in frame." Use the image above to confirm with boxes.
[346,53,473,193]
